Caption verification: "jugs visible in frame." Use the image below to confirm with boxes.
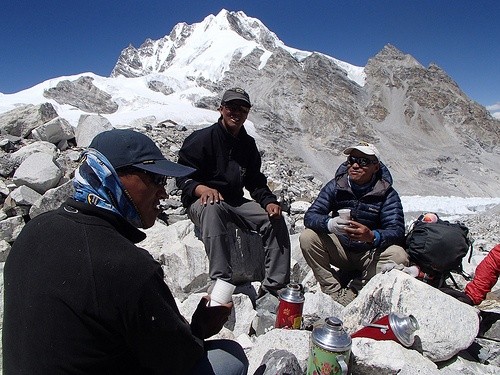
[275,282,305,330]
[351,313,420,349]
[306,316,352,375]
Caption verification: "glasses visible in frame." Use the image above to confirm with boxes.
[347,156,373,167]
[225,102,250,114]
[129,169,168,186]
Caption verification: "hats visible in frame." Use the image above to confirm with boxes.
[343,142,380,162]
[222,87,252,108]
[83,128,198,178]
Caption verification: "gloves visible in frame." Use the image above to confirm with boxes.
[328,217,354,236]
[440,287,473,306]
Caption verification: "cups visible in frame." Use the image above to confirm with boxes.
[337,209,351,220]
[205,277,236,307]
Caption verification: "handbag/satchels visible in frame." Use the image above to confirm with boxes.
[228,227,265,285]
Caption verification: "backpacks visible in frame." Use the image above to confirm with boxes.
[407,211,474,287]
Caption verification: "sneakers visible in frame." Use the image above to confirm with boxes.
[339,288,357,307]
[330,287,344,302]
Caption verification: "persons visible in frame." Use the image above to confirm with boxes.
[298,144,411,307]
[2,128,249,375]
[439,243,500,307]
[175,87,290,298]
